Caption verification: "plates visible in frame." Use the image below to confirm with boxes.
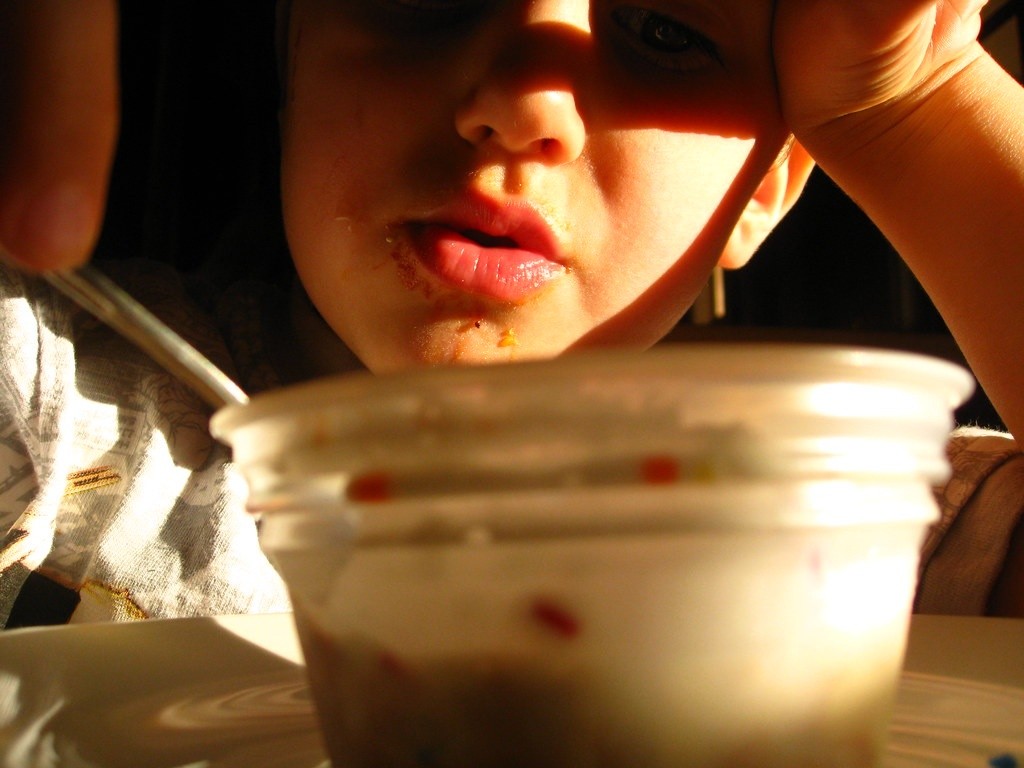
[0,612,1024,768]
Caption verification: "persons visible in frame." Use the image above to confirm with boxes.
[0,0,1024,634]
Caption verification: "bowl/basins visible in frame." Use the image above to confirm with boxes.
[208,345,973,768]
